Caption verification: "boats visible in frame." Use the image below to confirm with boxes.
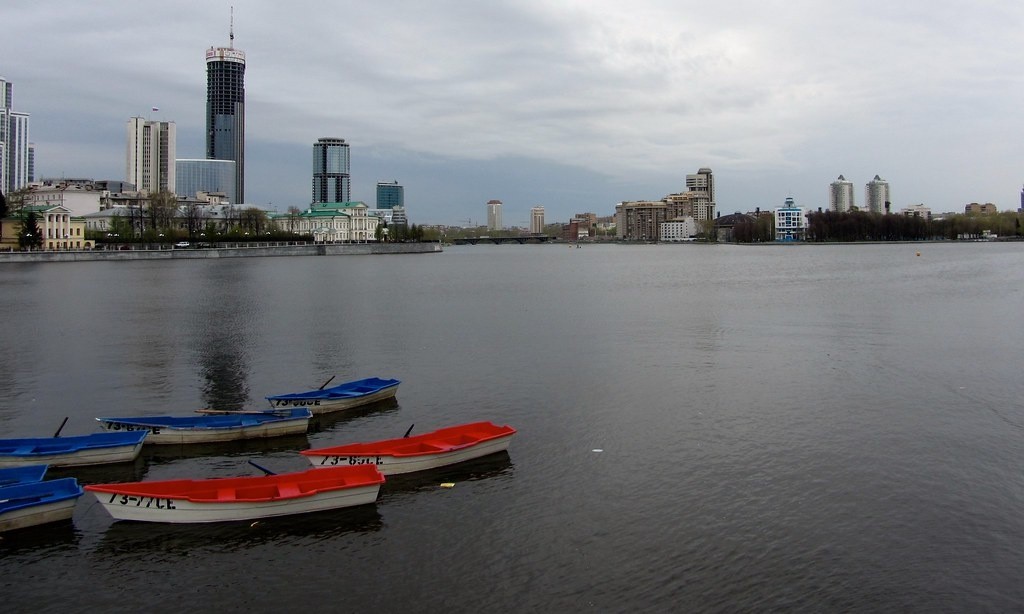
[298,423,516,488]
[0,431,149,534]
[264,376,402,424]
[96,406,313,451]
[83,463,386,528]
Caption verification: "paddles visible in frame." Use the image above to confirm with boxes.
[248,459,277,475]
[0,480,20,485]
[319,375,336,390]
[403,424,415,438]
[54,416,69,437]
[0,492,55,503]
[194,409,292,417]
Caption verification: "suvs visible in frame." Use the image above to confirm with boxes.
[175,241,189,248]
